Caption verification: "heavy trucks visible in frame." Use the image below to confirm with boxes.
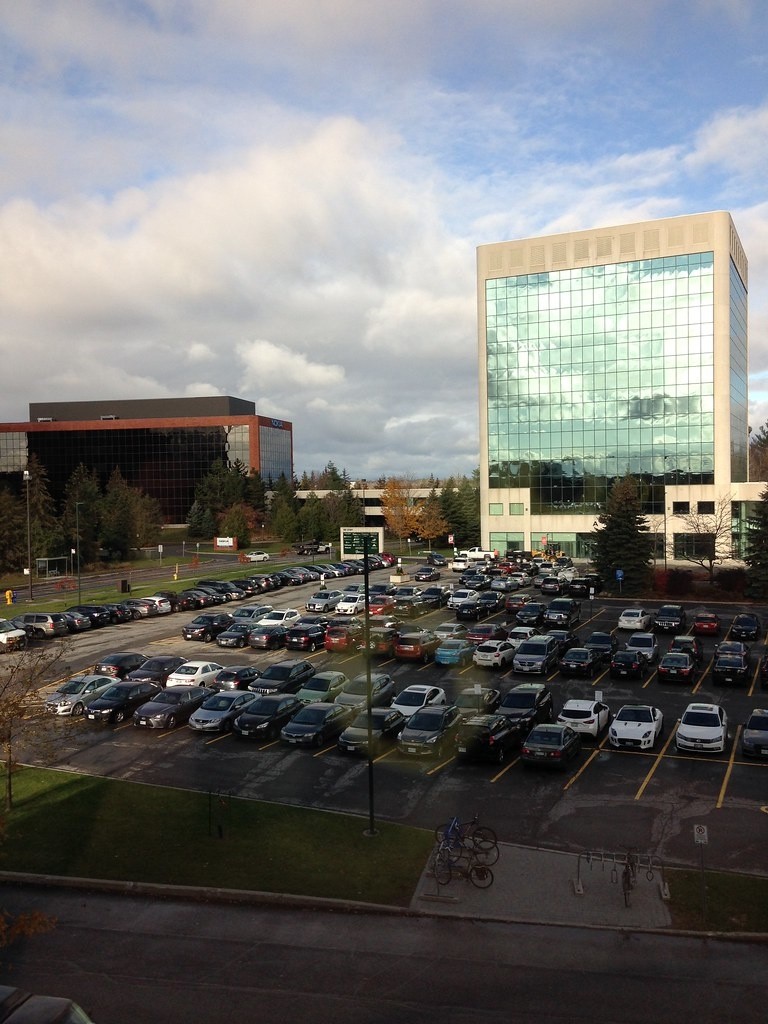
[292,542,332,556]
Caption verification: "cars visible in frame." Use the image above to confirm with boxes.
[693,613,721,635]
[657,652,698,686]
[556,699,611,742]
[84,680,163,723]
[458,687,502,718]
[395,703,467,761]
[760,652,768,688]
[610,649,648,679]
[189,690,264,734]
[710,641,751,687]
[45,674,123,716]
[280,702,353,749]
[608,704,664,750]
[337,705,406,755]
[389,684,446,724]
[233,691,306,740]
[243,551,271,563]
[669,636,703,665]
[1,547,617,706]
[617,608,651,632]
[133,683,216,730]
[520,723,584,772]
[742,707,768,762]
[731,612,761,641]
[626,632,659,664]
[298,670,351,705]
[675,703,728,754]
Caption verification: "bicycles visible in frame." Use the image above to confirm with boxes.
[431,811,500,889]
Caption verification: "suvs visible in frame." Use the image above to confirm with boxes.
[654,604,687,635]
[453,712,521,763]
[247,659,316,696]
[334,671,396,713]
[494,683,554,733]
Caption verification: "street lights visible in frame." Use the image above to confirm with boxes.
[23,470,34,601]
[74,500,87,606]
[362,535,378,836]
